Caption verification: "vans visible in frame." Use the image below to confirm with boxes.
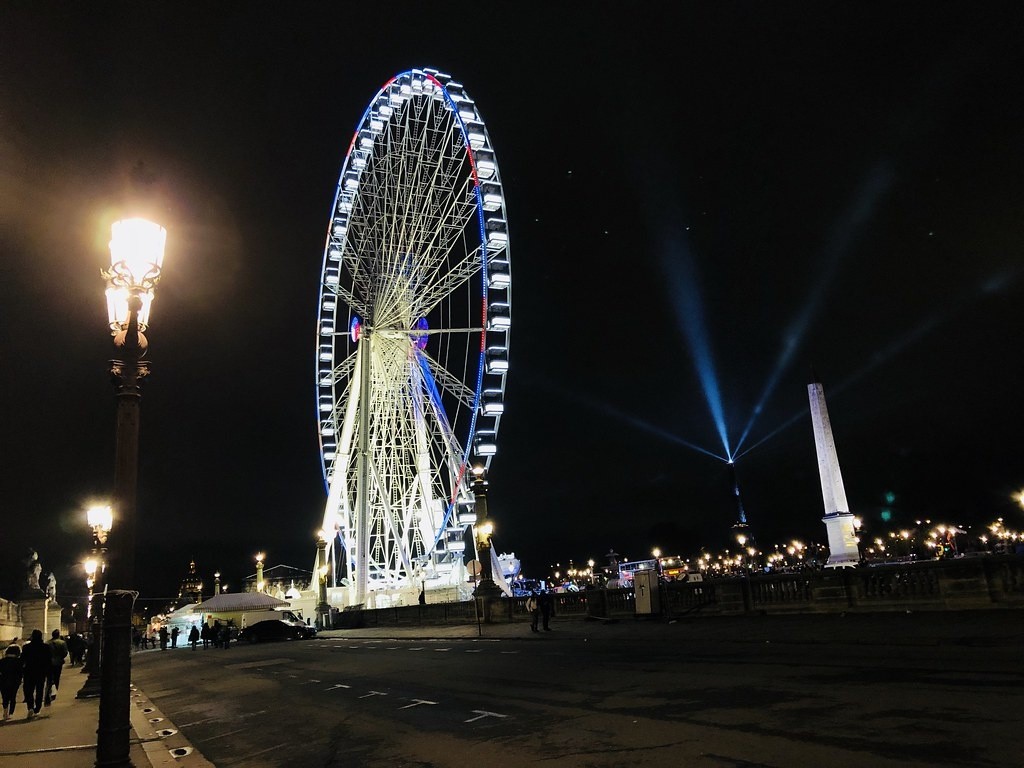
[241,610,307,629]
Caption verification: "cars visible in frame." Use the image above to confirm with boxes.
[237,619,317,644]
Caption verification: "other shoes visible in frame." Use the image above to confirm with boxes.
[3,709,12,720]
[27,709,34,720]
[34,705,41,714]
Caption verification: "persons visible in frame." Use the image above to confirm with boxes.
[188,620,231,650]
[0,628,88,719]
[141,626,181,650]
[526,590,552,632]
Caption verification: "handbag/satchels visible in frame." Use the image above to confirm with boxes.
[44,687,52,707]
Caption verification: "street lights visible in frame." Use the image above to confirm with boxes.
[314,529,332,614]
[95,216,166,768]
[74,498,114,700]
[420,571,425,605]
[471,463,504,598]
[652,547,661,574]
[198,585,202,604]
[214,572,220,597]
[589,559,595,585]
[733,521,747,564]
[255,552,266,594]
